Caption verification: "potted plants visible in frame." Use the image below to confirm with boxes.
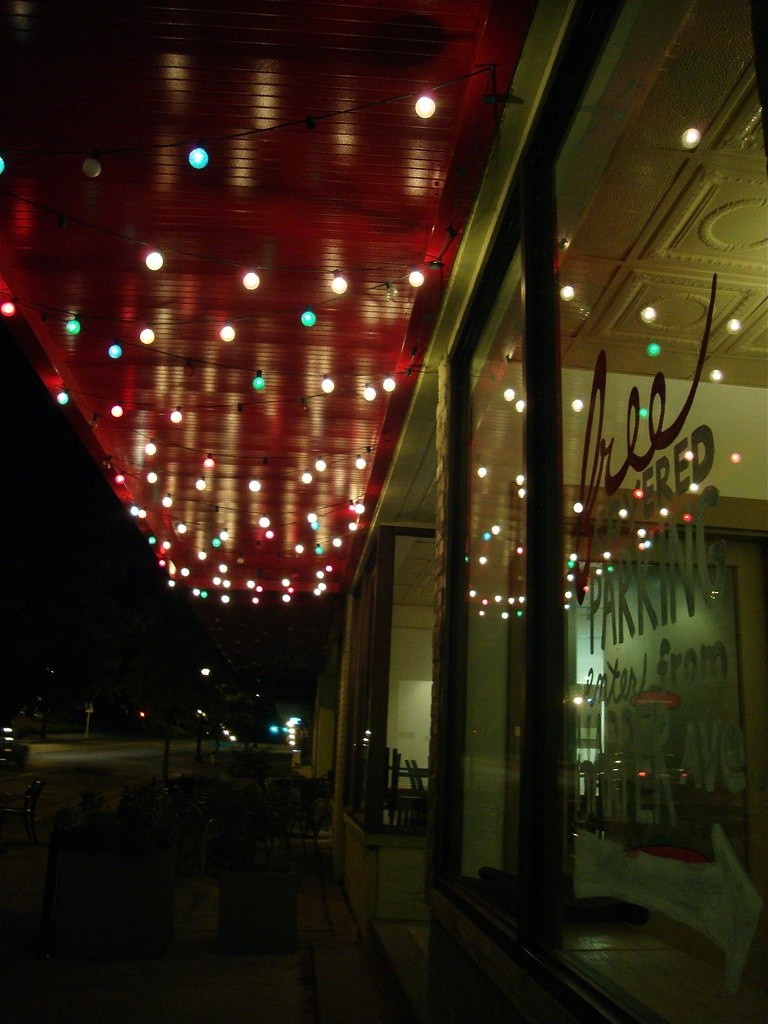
[53,772,309,959]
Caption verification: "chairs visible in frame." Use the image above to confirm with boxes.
[383,749,432,829]
[260,769,335,855]
[0,775,50,848]
